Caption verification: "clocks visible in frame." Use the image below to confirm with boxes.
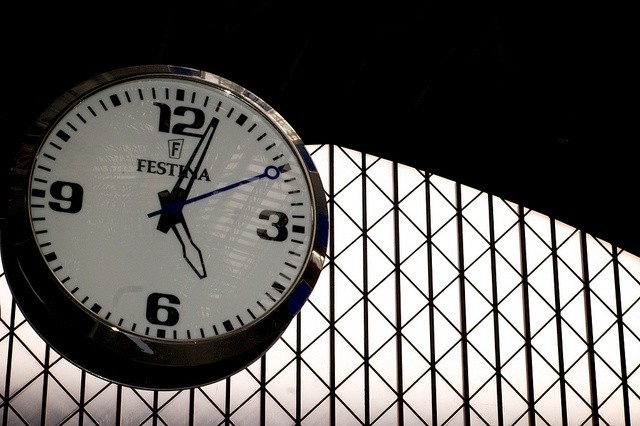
[0,63,329,391]
